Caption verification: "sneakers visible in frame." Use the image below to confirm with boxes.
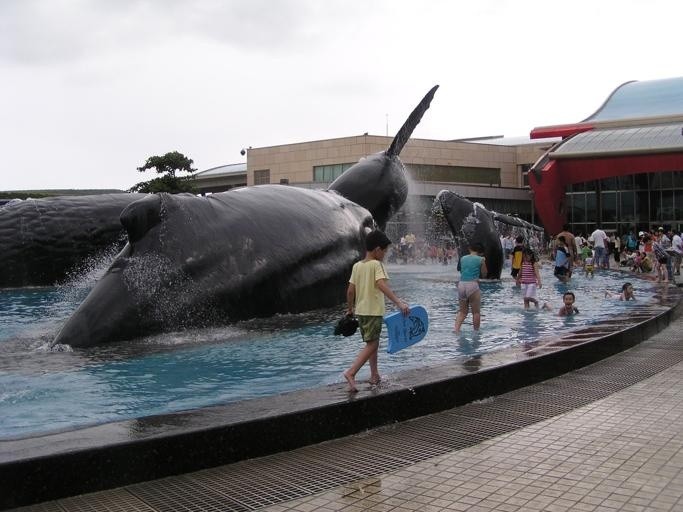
[334,314,360,337]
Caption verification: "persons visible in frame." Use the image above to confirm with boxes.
[593,283,636,301]
[451,241,488,335]
[516,248,542,311]
[541,292,579,316]
[388,223,683,288]
[343,230,409,393]
